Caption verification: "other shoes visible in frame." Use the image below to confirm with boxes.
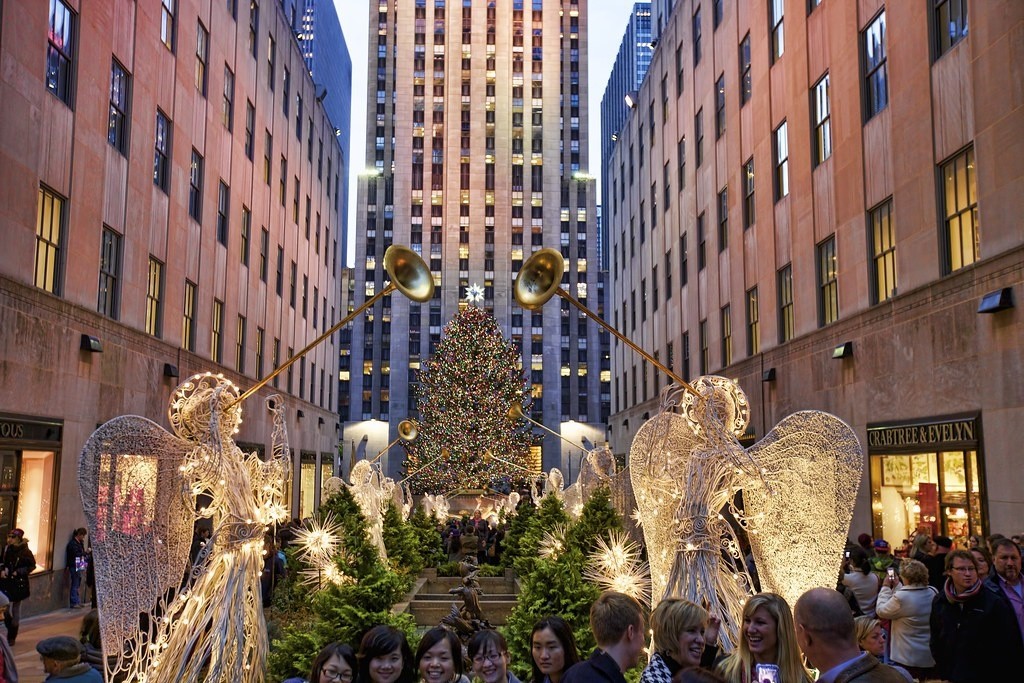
[74,603,87,608]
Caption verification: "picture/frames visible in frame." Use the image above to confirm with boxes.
[881,455,931,487]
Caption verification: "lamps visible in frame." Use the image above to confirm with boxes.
[607,425,613,430]
[625,90,639,108]
[612,130,620,140]
[79,333,103,353]
[830,341,852,358]
[336,423,341,430]
[316,83,328,101]
[976,286,1013,313]
[335,125,342,136]
[621,419,628,426]
[297,410,304,417]
[163,363,179,377]
[641,412,649,420]
[650,37,660,49]
[760,367,776,382]
[319,417,326,424]
[269,400,276,408]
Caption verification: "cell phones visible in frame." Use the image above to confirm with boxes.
[887,567,894,580]
[756,663,780,683]
[845,551,851,561]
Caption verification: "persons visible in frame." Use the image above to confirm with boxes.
[36,636,105,683]
[282,511,1023,683]
[0,528,37,645]
[66,527,97,609]
[0,591,19,683]
[192,520,210,565]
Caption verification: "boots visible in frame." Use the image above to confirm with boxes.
[3,611,19,645]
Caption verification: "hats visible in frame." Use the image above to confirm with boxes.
[933,536,952,549]
[874,539,889,551]
[36,636,84,659]
[8,528,24,539]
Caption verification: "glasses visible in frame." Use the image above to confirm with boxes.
[876,550,887,555]
[321,667,355,683]
[473,649,507,664]
[40,655,45,661]
[953,566,976,572]
[8,535,16,538]
